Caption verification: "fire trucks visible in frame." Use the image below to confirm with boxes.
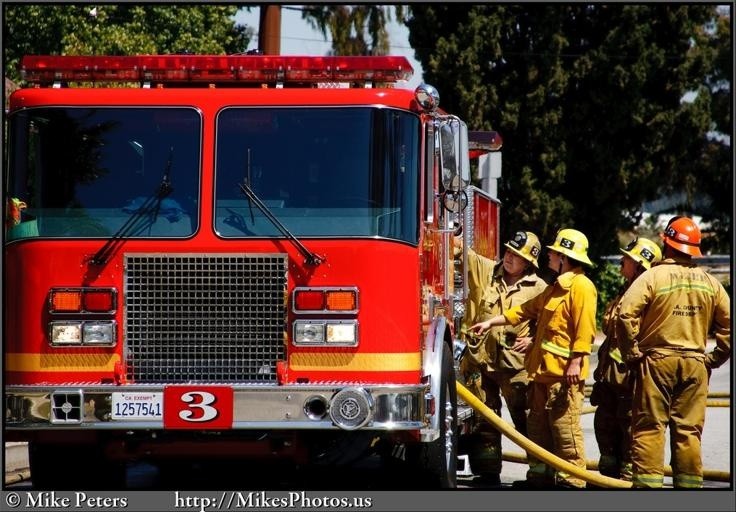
[0,48,509,491]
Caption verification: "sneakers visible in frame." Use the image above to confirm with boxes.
[472,474,501,485]
[512,480,548,491]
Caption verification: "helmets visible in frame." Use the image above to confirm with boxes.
[619,237,662,271]
[503,230,542,269]
[659,215,703,257]
[546,228,592,265]
